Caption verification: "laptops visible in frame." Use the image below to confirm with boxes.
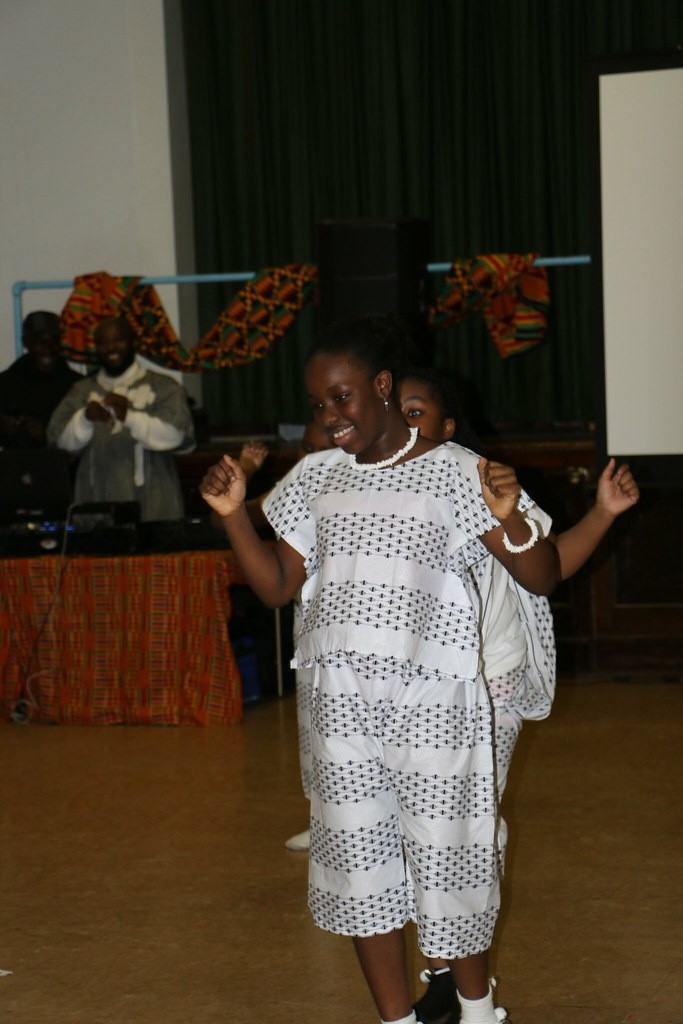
[0,448,75,505]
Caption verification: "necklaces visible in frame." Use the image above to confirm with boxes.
[349,428,418,470]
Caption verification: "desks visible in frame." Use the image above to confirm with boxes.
[0,519,282,728]
[182,419,619,642]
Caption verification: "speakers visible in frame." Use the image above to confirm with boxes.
[317,215,436,380]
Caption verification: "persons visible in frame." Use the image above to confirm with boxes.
[210,419,339,850]
[0,310,85,516]
[197,322,561,1024]
[44,317,198,550]
[400,368,641,1024]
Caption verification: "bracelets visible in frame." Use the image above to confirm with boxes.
[503,519,538,554]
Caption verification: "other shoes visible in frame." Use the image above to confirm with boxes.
[445,1002,462,1024]
[412,968,454,1024]
